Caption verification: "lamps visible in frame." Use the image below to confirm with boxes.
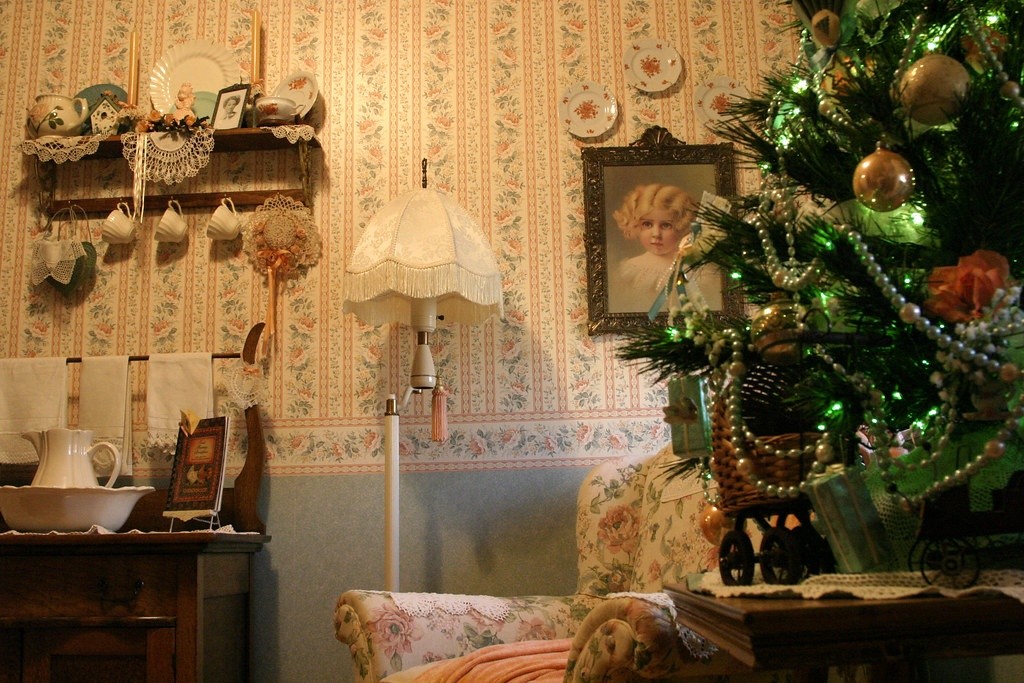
[342,157,505,591]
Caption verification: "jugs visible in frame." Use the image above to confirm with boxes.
[25,93,88,139]
[21,428,121,489]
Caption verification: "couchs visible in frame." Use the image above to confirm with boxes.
[333,437,814,683]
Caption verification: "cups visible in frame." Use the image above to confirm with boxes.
[154,200,188,243]
[255,95,306,125]
[102,202,136,244]
[206,198,242,241]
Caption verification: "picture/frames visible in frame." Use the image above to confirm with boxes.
[580,125,744,336]
[211,83,252,130]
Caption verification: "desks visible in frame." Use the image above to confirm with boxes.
[657,569,1024,683]
[1,402,274,683]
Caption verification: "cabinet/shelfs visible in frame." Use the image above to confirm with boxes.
[20,113,313,216]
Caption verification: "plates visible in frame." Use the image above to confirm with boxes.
[150,40,236,120]
[622,39,682,91]
[560,81,618,137]
[693,76,751,132]
[275,70,318,119]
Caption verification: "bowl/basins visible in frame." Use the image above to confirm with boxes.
[0,484,157,533]
